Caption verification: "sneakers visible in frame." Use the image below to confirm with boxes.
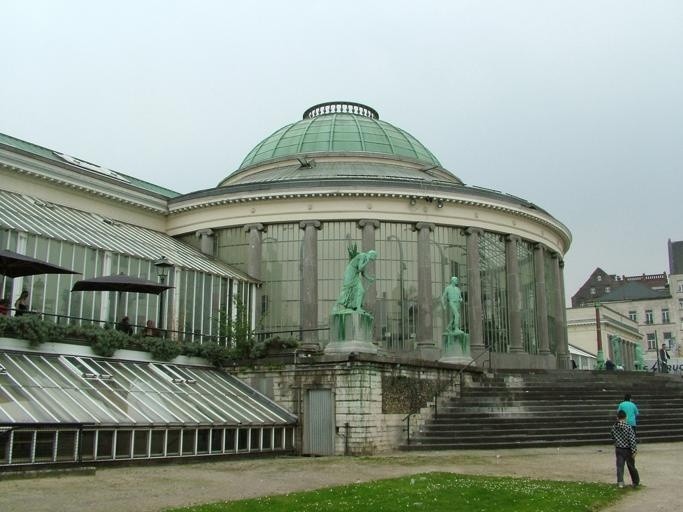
[618,482,639,489]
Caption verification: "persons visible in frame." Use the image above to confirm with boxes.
[115,316,133,337]
[616,390,640,443]
[11,290,28,316]
[659,342,670,372]
[440,274,464,334]
[328,248,378,313]
[608,409,645,489]
[0,299,8,316]
[139,319,154,338]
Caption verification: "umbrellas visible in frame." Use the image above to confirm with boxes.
[69,271,176,330]
[0,247,83,281]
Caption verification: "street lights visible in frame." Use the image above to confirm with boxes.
[589,294,605,369]
[152,253,176,339]
[382,232,479,354]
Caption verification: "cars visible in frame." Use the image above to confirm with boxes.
[466,315,509,355]
